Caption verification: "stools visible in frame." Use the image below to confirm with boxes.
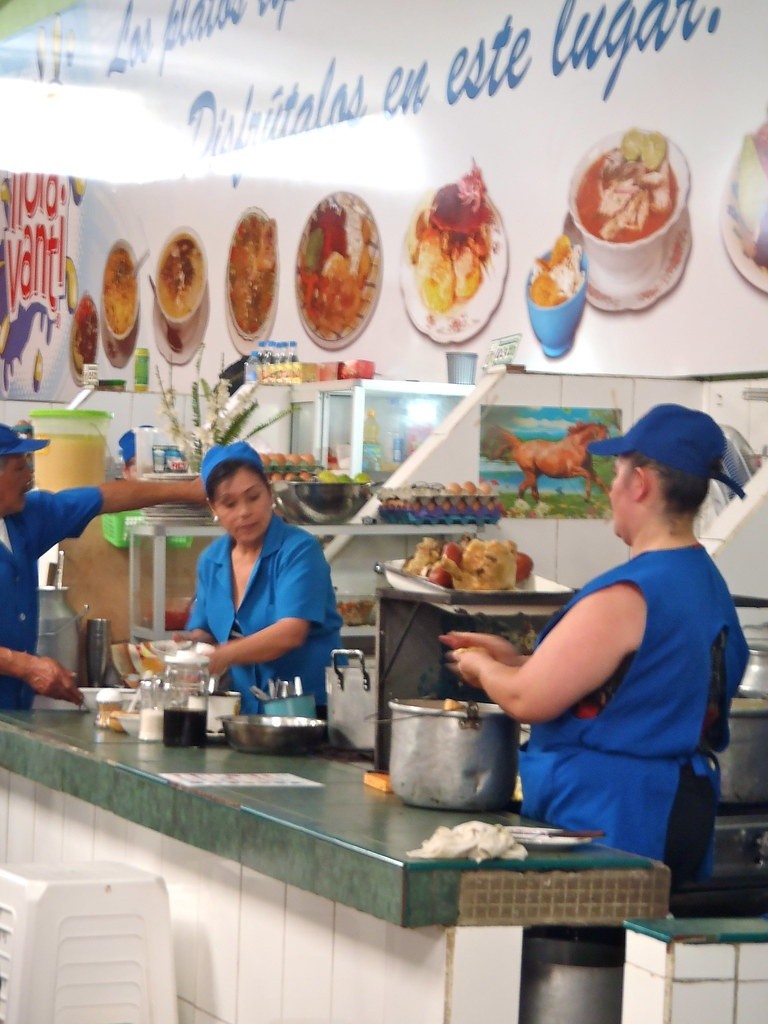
[0,862,177,1024]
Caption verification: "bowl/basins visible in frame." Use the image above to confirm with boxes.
[216,714,327,755]
[117,715,139,736]
[99,128,692,357]
[77,685,136,711]
[149,639,215,660]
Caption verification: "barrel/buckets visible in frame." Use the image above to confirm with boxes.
[37,585,78,675]
[28,410,114,492]
[364,697,522,811]
[445,352,478,386]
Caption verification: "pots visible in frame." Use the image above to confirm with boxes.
[269,480,382,523]
[707,620,768,808]
[324,648,375,751]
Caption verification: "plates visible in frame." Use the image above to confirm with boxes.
[140,470,211,526]
[720,152,768,294]
[67,186,691,387]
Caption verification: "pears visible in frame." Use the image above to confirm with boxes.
[317,470,372,484]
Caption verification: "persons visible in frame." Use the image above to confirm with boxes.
[171,442,347,718]
[0,423,209,707]
[119,425,153,480]
[440,403,746,906]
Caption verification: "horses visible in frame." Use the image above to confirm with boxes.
[482,419,615,504]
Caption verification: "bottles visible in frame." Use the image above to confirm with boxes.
[392,431,405,462]
[365,410,377,443]
[135,424,155,475]
[94,689,123,728]
[139,678,164,743]
[163,651,210,746]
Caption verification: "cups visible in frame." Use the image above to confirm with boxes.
[188,691,241,737]
[263,694,316,718]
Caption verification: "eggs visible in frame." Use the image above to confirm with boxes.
[380,481,495,511]
[258,451,315,480]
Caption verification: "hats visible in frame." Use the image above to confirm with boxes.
[0,422,49,455]
[587,404,746,499]
[201,441,264,495]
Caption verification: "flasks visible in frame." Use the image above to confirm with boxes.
[86,618,111,686]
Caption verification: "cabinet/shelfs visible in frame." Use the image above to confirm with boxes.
[129,523,477,646]
[290,380,482,485]
